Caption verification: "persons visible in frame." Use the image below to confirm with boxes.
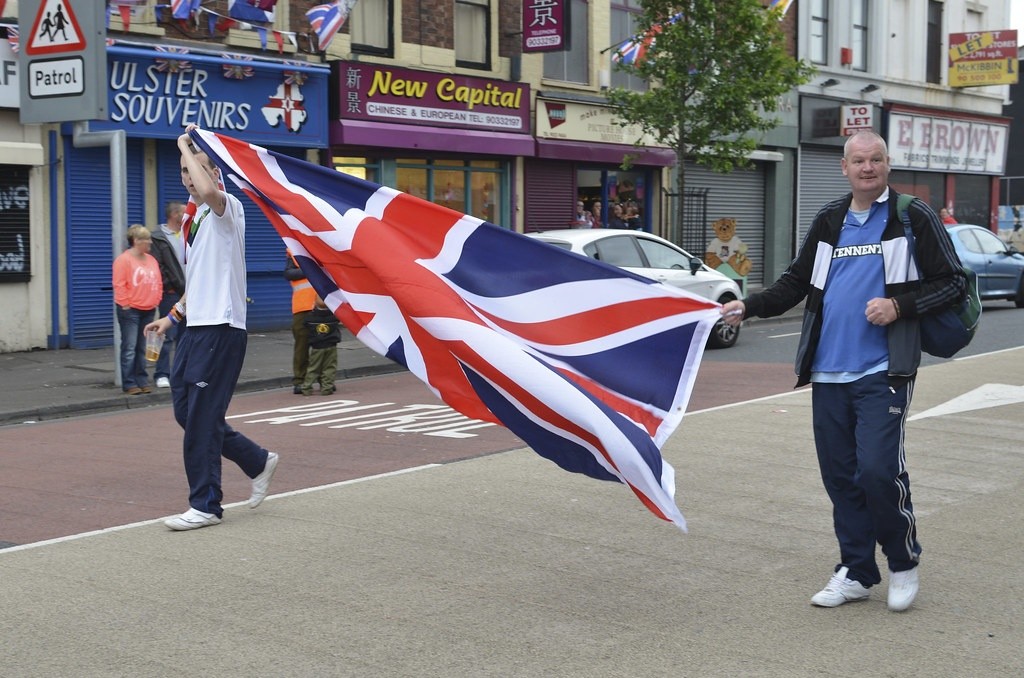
[301,296,341,396]
[285,248,337,394]
[144,124,278,531]
[112,224,163,396]
[148,203,186,389]
[718,129,969,611]
[1008,224,1024,255]
[577,199,641,231]
[940,208,958,224]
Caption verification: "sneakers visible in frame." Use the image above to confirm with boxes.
[164,507,222,530]
[886,565,919,612]
[809,566,871,608]
[248,452,279,509]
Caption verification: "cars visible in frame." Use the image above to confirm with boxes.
[522,228,743,348]
[941,223,1024,308]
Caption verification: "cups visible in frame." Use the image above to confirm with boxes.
[145,330,166,362]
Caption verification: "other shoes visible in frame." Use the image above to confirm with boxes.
[125,387,152,395]
[294,384,303,393]
[332,384,336,390]
[155,376,170,388]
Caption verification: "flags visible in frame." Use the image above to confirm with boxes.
[229,0,276,22]
[170,0,201,17]
[736,0,793,55]
[612,12,699,75]
[306,0,357,51]
[187,128,742,534]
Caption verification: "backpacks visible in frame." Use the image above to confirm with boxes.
[896,192,981,358]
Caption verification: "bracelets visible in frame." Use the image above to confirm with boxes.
[891,296,899,320]
[167,302,186,328]
